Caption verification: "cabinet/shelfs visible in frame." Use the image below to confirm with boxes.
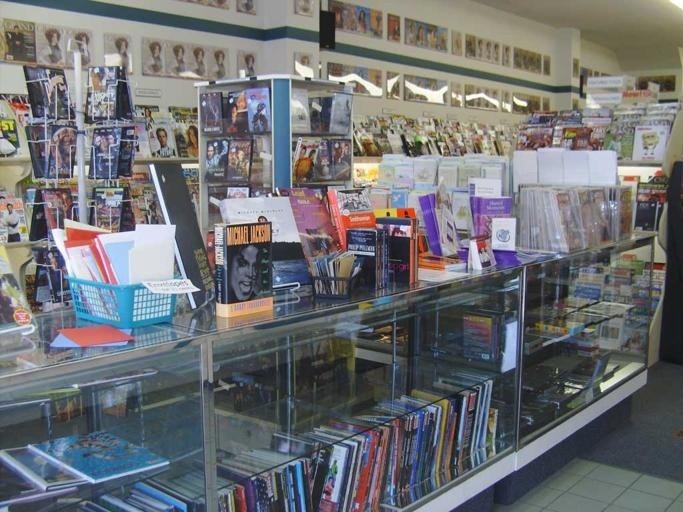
[0,230,657,512]
[616,108,683,367]
[193,73,355,251]
[1,155,383,300]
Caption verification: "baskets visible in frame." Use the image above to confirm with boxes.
[71,320,178,360]
[68,275,178,327]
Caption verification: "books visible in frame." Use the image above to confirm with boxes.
[170,258,668,512]
[214,179,518,319]
[0,429,205,511]
[290,91,680,255]
[1,64,274,319]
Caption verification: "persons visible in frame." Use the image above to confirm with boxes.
[334,1,550,75]
[0,17,263,81]
